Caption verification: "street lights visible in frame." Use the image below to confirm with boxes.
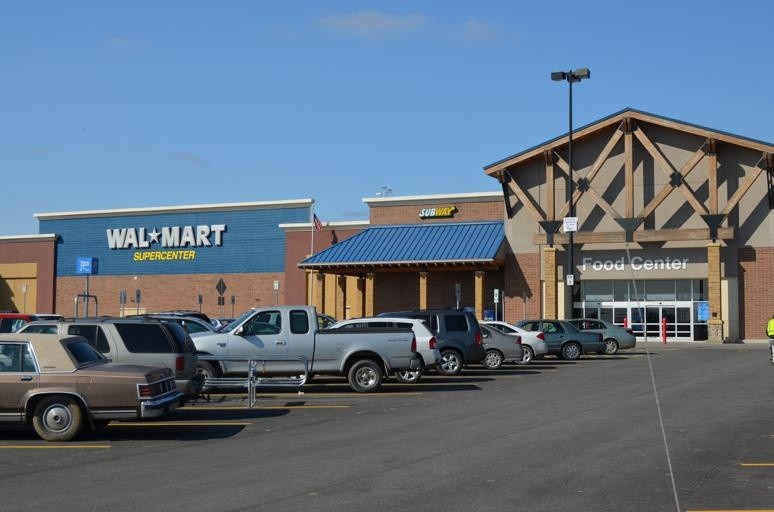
[550,68,591,319]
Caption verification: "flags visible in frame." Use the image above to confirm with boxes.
[314,212,322,232]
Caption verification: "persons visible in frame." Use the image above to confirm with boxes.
[765,313,774,366]
